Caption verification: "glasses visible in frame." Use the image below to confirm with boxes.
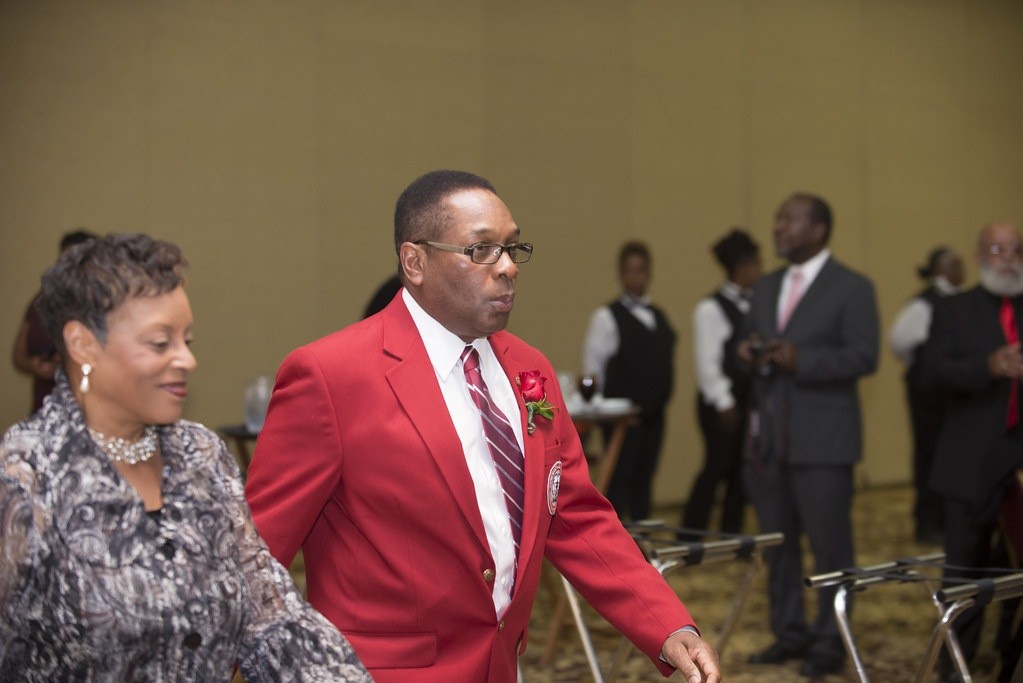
[398,238,535,278]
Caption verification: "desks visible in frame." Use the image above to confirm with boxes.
[538,409,640,672]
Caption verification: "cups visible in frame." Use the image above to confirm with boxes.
[578,372,599,407]
[246,375,272,434]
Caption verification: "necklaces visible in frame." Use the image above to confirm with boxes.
[87,424,159,465]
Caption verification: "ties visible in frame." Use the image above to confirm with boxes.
[776,270,803,333]
[997,294,1021,427]
[458,345,527,603]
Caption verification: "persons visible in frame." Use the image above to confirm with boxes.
[577,241,677,538]
[920,217,1023,683]
[890,247,968,540]
[674,227,758,542]
[736,188,880,677]
[244,170,722,683]
[0,232,376,683]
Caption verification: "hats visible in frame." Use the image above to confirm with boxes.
[711,227,761,267]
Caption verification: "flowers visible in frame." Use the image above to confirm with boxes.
[518,368,558,424]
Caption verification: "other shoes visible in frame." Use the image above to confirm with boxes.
[798,650,843,678]
[746,639,804,666]
